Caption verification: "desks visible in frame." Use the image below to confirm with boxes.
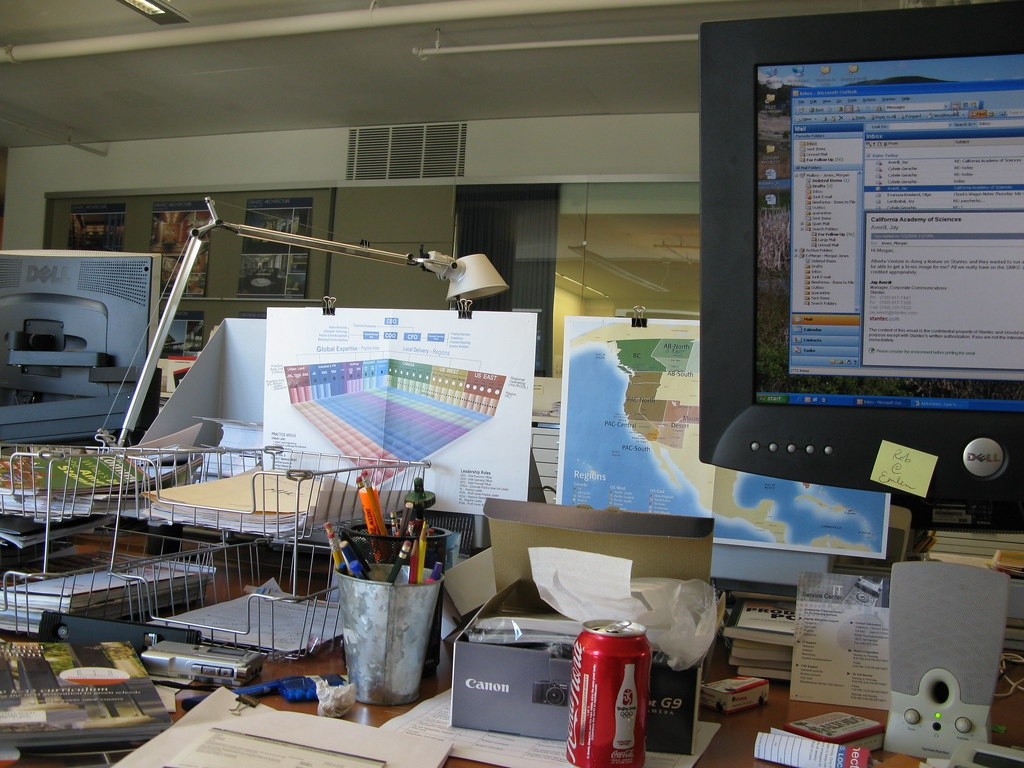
[0,519,1024,768]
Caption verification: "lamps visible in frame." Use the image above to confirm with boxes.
[99,196,512,450]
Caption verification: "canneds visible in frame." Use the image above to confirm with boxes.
[566,618,653,768]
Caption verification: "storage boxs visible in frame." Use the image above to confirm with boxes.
[452,496,714,755]
[166,355,198,393]
[701,676,770,714]
[785,711,886,751]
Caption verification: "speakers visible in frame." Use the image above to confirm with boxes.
[881,558,1010,761]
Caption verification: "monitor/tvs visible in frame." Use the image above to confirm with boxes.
[0,249,161,447]
[697,5,1024,506]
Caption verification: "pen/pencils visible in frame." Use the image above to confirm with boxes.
[322,470,443,584]
[281,654,438,701]
[182,674,305,713]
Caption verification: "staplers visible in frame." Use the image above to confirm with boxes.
[38,609,202,656]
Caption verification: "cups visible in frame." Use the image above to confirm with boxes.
[334,564,445,705]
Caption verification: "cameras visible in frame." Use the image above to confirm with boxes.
[139,640,265,688]
